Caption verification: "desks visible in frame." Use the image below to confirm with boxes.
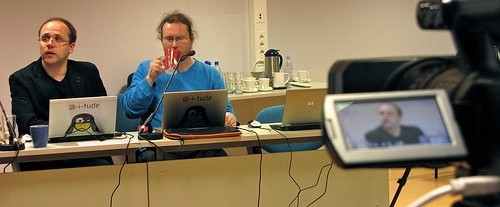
[0,130,389,207]
[225,78,334,123]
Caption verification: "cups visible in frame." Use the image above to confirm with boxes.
[222,71,243,94]
[1,115,16,145]
[255,78,269,89]
[30,125,49,148]
[244,80,259,90]
[298,71,309,81]
[273,72,290,86]
[241,78,256,87]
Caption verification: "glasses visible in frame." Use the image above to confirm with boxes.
[38,35,71,46]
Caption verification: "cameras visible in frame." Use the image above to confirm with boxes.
[322,0,499,168]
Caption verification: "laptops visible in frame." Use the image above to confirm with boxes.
[46,97,118,141]
[270,87,328,132]
[151,87,229,131]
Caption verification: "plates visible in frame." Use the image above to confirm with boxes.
[293,77,312,83]
[242,89,258,93]
[259,87,273,91]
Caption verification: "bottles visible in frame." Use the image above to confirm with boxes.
[283,55,293,82]
[204,60,211,66]
[214,61,221,76]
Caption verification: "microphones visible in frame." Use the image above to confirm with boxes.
[137,49,196,139]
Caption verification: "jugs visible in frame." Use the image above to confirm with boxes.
[264,49,283,87]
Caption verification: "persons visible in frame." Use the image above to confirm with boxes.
[363,102,427,147]
[121,11,236,163]
[8,17,114,172]
